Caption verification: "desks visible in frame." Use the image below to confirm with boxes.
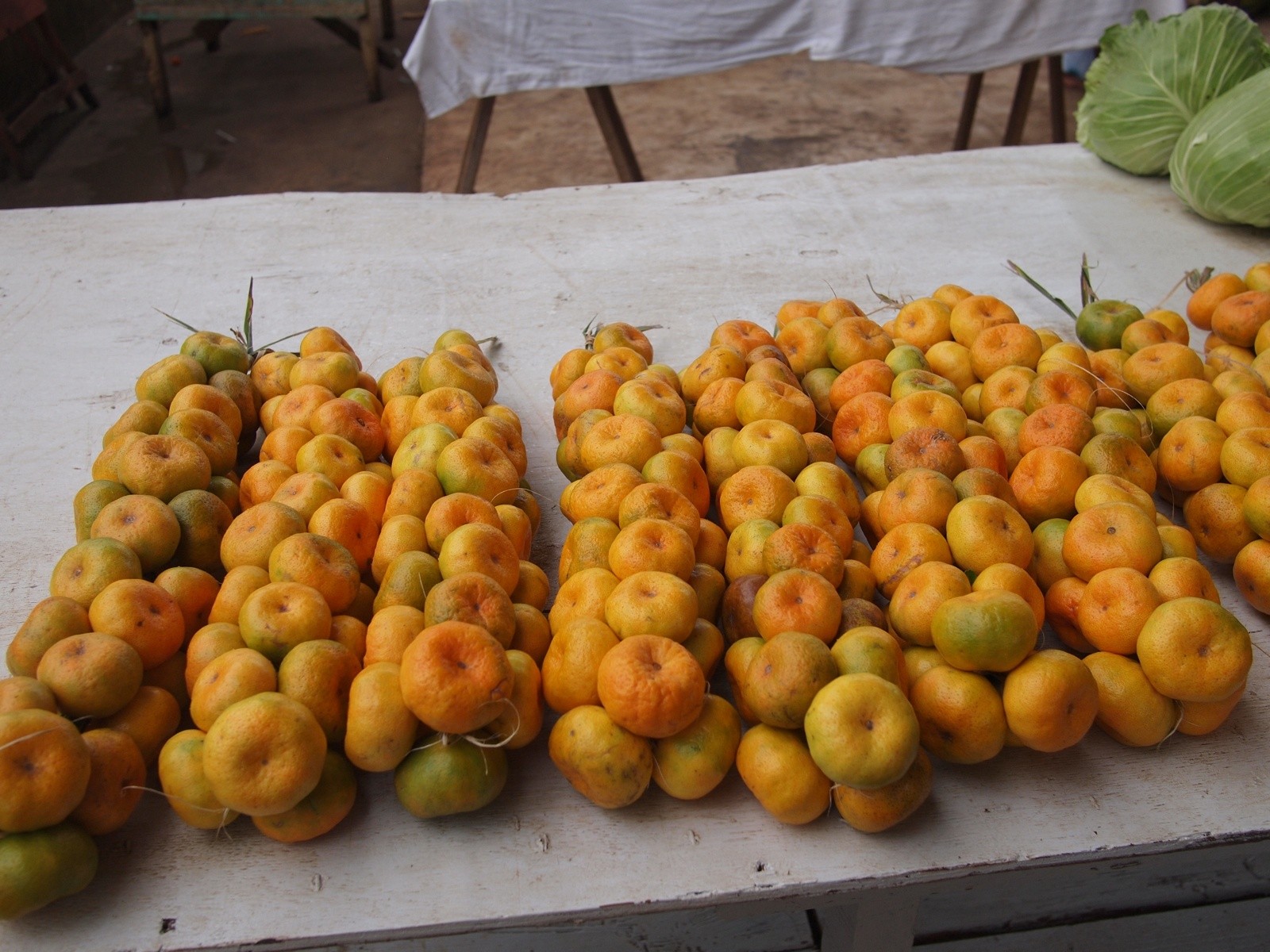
[2,140,1270,952]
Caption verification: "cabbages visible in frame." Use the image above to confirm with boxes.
[1075,6,1270,234]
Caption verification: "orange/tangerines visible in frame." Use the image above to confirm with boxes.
[0,268,1270,912]
